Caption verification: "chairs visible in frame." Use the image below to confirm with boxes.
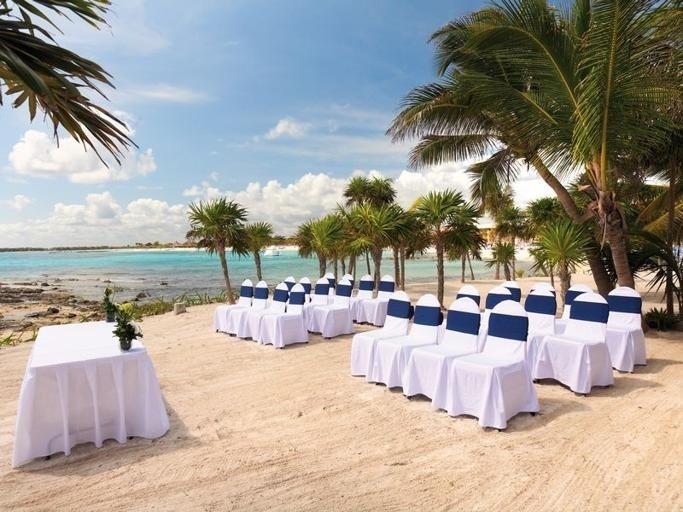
[529,283,557,299]
[448,286,481,314]
[522,289,558,381]
[501,280,522,303]
[402,296,482,411]
[604,285,649,373]
[445,302,540,430]
[555,283,593,334]
[481,288,513,342]
[216,271,396,349]
[349,290,413,377]
[535,291,616,395]
[370,292,442,391]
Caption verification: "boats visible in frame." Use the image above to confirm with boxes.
[264,246,280,257]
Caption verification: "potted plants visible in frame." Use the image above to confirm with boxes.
[103,281,116,323]
[110,302,142,350]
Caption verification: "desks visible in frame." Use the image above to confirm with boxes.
[10,323,169,472]
